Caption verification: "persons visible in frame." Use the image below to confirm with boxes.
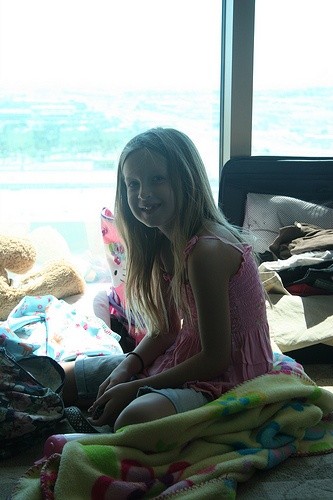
[51,127,272,431]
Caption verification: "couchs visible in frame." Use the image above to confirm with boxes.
[216,155,333,366]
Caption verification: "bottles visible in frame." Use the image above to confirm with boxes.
[34,433,96,466]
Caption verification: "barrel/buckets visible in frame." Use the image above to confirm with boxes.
[100,208,127,288]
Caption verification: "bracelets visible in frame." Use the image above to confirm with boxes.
[126,352,144,373]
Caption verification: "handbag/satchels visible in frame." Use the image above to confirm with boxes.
[0,342,100,460]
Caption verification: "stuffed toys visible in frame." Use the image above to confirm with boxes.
[0,233,84,325]
